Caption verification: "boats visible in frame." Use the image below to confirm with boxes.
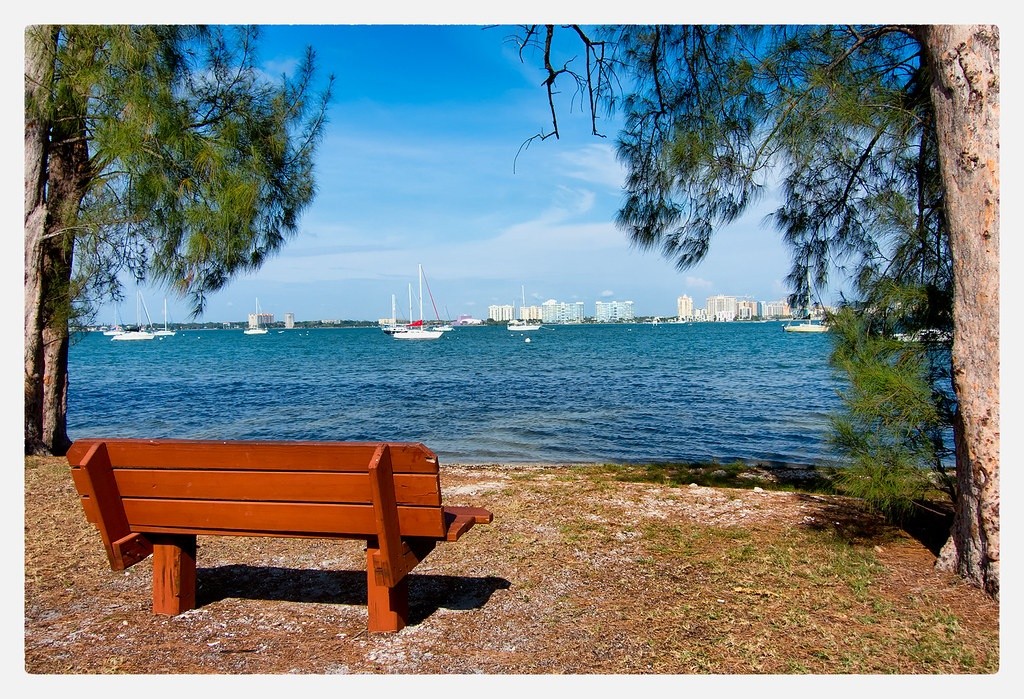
[890,330,952,344]
[434,325,453,332]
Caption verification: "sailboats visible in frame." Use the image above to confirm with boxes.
[244,297,268,335]
[507,285,541,331]
[112,291,154,340]
[394,263,444,340]
[783,271,830,332]
[103,300,127,336]
[382,283,417,335]
[155,299,175,337]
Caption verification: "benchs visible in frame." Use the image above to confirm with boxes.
[67,440,494,632]
